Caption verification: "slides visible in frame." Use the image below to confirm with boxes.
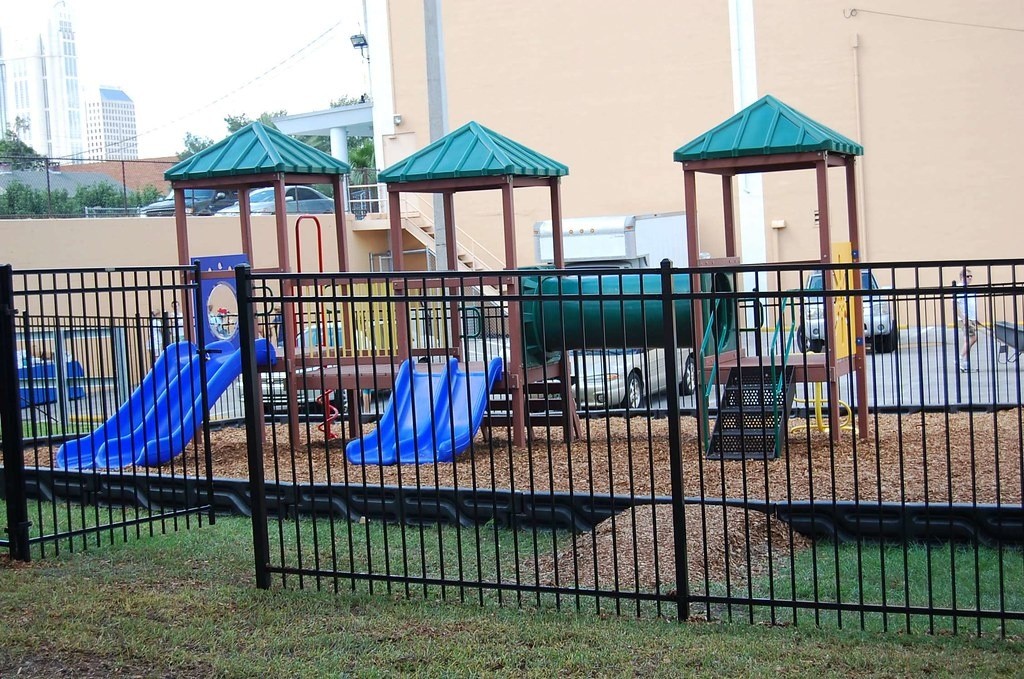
[345,356,504,466]
[56,337,278,470]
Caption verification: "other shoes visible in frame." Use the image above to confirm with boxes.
[954,361,967,372]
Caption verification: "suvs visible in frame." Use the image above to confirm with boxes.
[796,272,899,355]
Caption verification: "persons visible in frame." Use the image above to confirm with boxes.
[956,270,979,373]
[169,301,185,343]
[146,310,163,363]
[268,305,284,348]
[208,304,228,337]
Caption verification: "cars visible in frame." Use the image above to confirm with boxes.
[546,343,700,412]
[410,336,510,372]
[239,322,391,414]
[140,182,236,217]
[212,185,335,216]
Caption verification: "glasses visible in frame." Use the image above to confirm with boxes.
[966,275,972,278]
